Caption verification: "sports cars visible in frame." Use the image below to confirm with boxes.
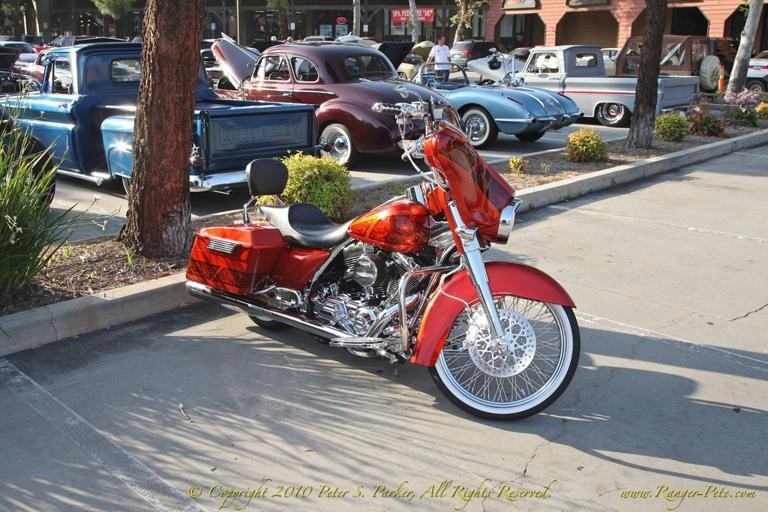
[367,41,584,148]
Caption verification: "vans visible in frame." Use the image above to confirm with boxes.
[1,40,34,90]
[603,47,620,76]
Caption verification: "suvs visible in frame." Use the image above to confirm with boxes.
[616,35,720,91]
[449,40,508,67]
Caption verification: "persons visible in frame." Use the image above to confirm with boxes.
[426,37,453,83]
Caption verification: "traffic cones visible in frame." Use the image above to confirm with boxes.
[716,65,726,94]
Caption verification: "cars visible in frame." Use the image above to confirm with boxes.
[747,51,768,91]
[398,54,423,79]
[211,40,461,167]
[11,48,71,89]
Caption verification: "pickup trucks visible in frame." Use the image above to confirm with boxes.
[0,43,332,197]
[469,45,699,127]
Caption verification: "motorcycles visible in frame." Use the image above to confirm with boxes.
[186,86,582,421]
